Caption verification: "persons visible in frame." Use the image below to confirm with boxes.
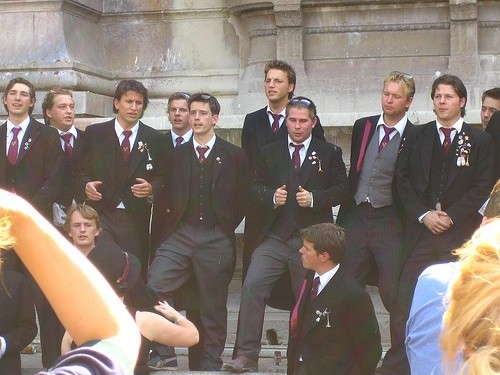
[0,60,500,375]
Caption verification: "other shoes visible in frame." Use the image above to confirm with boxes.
[146,355,178,370]
[224,356,258,372]
[376,366,395,375]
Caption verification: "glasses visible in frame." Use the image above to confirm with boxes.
[289,99,311,107]
[200,93,211,100]
[391,71,413,80]
[183,93,190,99]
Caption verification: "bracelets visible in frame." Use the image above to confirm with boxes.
[176,316,186,324]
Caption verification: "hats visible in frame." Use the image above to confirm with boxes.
[87,243,141,297]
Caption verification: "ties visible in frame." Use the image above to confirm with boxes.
[58,133,73,156]
[290,143,304,168]
[269,111,282,135]
[378,125,395,152]
[175,137,183,146]
[8,127,20,165]
[196,146,208,162]
[121,131,132,156]
[440,127,455,148]
[310,277,320,298]
